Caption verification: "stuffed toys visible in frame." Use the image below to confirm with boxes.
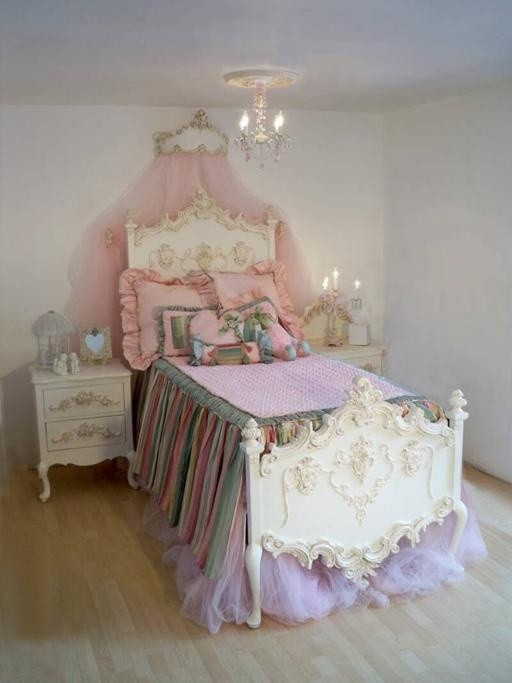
[251,307,311,362]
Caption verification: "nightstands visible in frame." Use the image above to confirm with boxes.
[305,335,388,377]
[27,358,141,503]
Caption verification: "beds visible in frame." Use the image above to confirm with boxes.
[124,185,470,629]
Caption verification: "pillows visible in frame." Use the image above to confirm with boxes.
[119,259,310,371]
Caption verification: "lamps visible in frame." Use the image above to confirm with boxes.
[223,69,300,162]
[322,267,361,347]
